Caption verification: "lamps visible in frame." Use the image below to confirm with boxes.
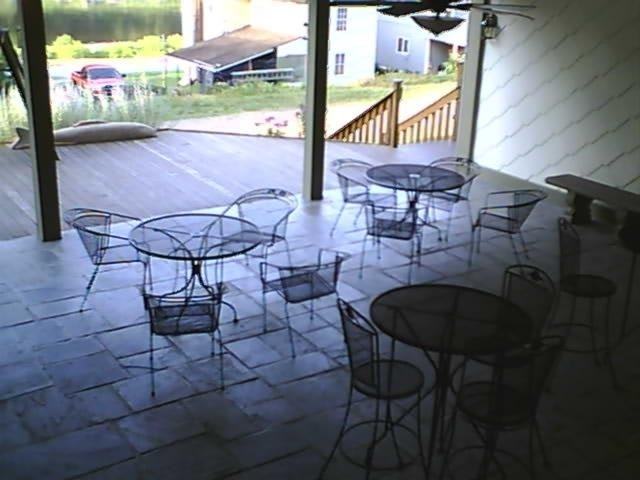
[410,10,466,35]
[482,15,498,39]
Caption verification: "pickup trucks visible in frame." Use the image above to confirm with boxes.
[71,62,129,97]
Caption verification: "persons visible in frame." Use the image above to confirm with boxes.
[91,91,101,113]
[60,85,71,104]
[104,82,113,100]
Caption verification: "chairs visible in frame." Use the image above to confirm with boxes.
[466,185,549,266]
[330,157,425,283]
[260,246,351,359]
[336,296,424,462]
[414,155,482,244]
[144,281,229,396]
[62,205,153,313]
[437,262,556,474]
[198,187,299,292]
[534,214,616,380]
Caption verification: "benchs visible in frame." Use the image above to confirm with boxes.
[543,173,639,246]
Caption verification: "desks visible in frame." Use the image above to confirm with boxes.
[369,282,535,477]
[364,163,466,256]
[127,212,265,324]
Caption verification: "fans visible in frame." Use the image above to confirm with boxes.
[330,0,536,22]
